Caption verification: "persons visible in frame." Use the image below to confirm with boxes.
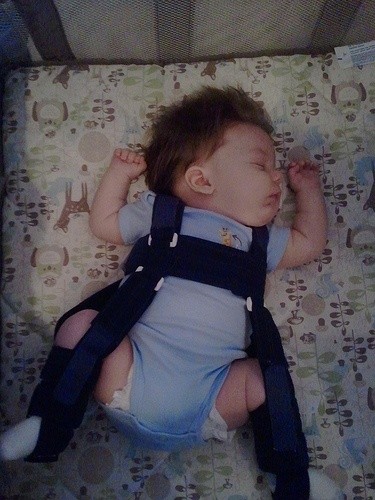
[1,87,340,500]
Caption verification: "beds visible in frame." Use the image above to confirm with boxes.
[0,0,374,499]
